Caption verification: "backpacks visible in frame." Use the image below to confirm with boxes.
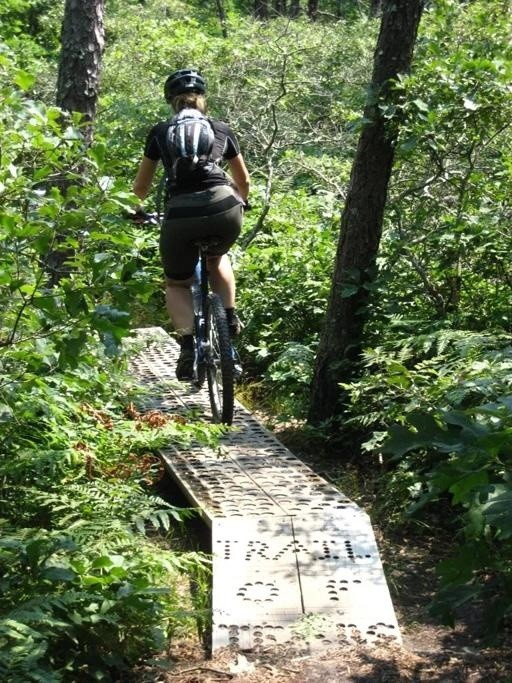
[166,107,215,182]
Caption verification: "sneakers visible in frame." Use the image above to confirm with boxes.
[229,323,240,336]
[176,349,195,380]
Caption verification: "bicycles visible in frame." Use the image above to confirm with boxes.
[126,203,252,427]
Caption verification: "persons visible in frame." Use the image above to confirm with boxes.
[133,63,256,380]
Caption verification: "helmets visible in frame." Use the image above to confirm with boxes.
[165,68,206,104]
